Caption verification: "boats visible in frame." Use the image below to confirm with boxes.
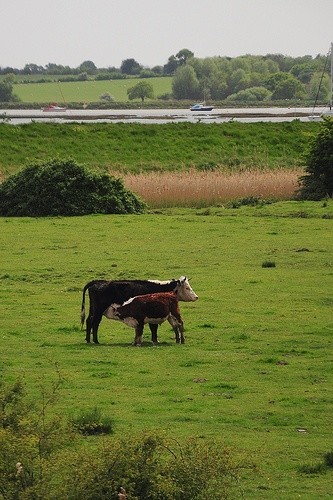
[308,110,333,122]
[42,103,66,112]
[190,103,214,111]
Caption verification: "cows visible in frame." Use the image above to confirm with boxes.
[102,292,185,347]
[79,275,199,344]
